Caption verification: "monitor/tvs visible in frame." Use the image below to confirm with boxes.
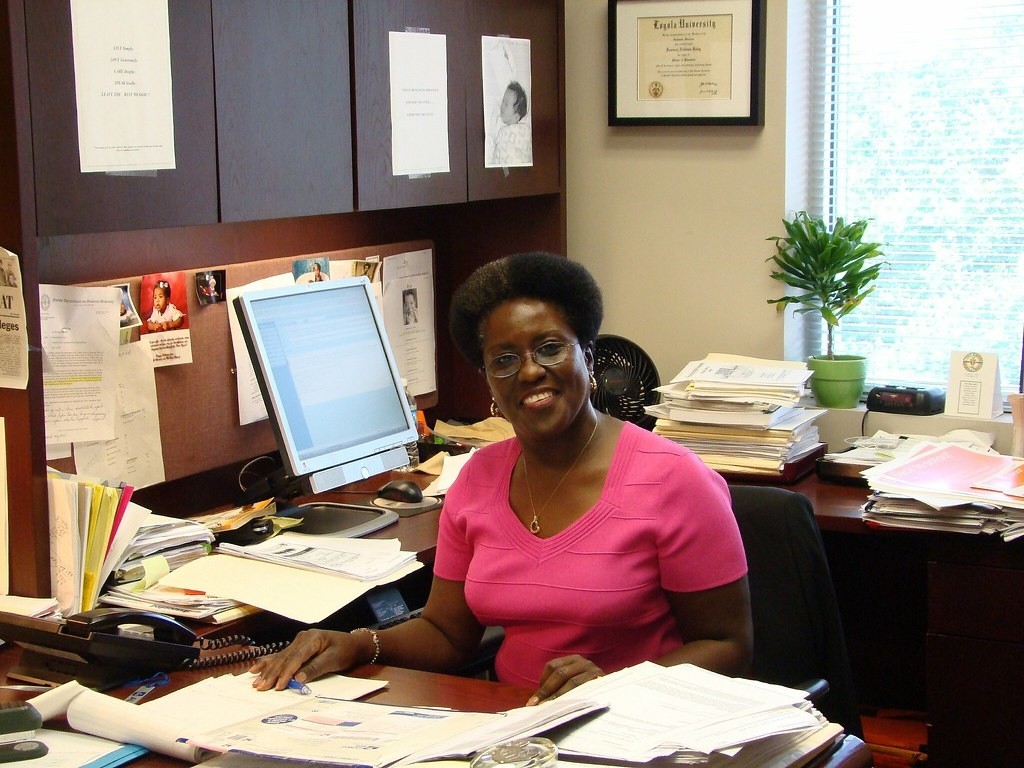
[234,274,419,539]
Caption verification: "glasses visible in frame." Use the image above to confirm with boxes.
[479,342,574,378]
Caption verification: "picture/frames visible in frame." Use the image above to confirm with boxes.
[608,0,768,127]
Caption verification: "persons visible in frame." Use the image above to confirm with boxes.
[249,254,754,706]
[361,263,371,283]
[206,279,219,296]
[308,263,323,283]
[120,299,138,327]
[494,82,533,165]
[146,280,185,331]
[404,293,418,325]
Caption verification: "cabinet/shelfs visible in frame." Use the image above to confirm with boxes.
[0,0,567,243]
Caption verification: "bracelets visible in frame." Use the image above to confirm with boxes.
[351,628,380,665]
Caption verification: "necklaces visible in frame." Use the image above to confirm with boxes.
[521,410,597,533]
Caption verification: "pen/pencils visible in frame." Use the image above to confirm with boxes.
[288,679,312,695]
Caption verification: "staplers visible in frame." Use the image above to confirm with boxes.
[0,701,48,763]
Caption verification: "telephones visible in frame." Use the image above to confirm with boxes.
[0,608,201,690]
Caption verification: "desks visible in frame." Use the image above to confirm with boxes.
[0,418,1024,768]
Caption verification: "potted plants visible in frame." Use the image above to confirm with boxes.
[764,211,893,409]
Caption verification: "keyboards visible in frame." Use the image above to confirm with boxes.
[365,607,505,648]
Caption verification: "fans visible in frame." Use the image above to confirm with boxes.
[589,333,662,433]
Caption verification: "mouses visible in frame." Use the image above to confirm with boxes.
[376,480,423,503]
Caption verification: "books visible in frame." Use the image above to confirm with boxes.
[184,497,276,533]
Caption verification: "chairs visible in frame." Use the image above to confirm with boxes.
[726,485,872,744]
